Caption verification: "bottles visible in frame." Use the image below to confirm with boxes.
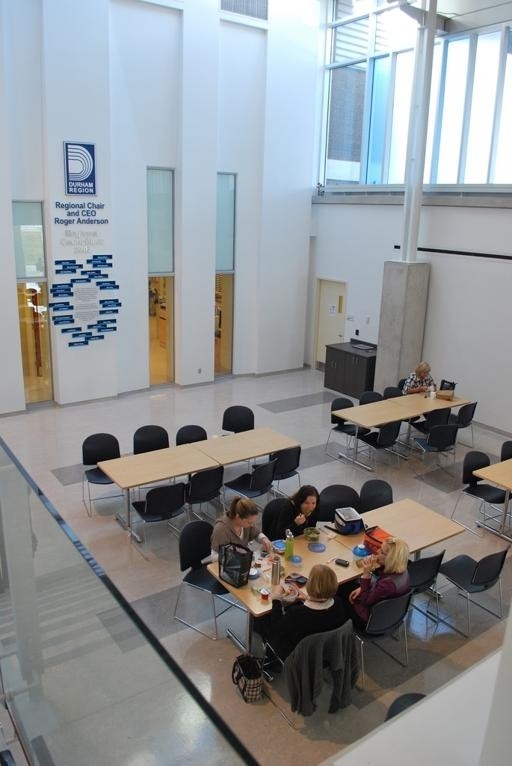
[355,554,378,569]
[353,545,369,561]
[288,556,303,578]
[284,530,294,561]
[271,555,280,585]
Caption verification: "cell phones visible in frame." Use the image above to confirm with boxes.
[260,550,270,558]
[336,559,349,566]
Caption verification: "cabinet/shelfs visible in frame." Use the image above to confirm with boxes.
[322,337,378,400]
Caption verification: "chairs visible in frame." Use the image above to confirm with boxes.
[83,433,121,517]
[175,521,248,639]
[426,546,510,638]
[130,485,190,542]
[361,480,393,513]
[450,451,512,538]
[355,589,415,691]
[176,425,208,446]
[501,440,512,461]
[224,458,276,512]
[222,406,254,433]
[318,485,359,521]
[262,619,354,704]
[408,549,446,625]
[185,466,225,523]
[252,446,300,500]
[326,379,478,472]
[261,500,300,541]
[134,426,168,455]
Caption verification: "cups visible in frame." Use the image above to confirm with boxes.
[261,589,270,602]
[430,392,436,399]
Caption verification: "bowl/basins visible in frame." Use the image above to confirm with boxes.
[279,585,300,604]
[303,526,322,541]
[272,540,285,555]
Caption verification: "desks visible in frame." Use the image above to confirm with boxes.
[98,426,301,543]
[473,458,512,543]
[206,498,466,617]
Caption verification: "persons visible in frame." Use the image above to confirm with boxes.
[271,565,354,663]
[211,495,273,561]
[270,485,321,537]
[401,361,437,395]
[333,536,410,628]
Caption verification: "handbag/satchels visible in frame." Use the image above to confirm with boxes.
[229,652,265,704]
[440,379,456,391]
[218,542,254,589]
